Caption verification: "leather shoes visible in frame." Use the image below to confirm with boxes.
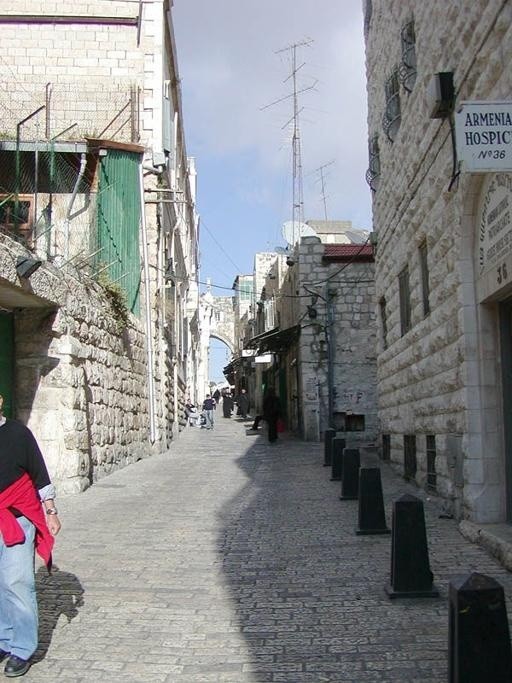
[0,649,33,678]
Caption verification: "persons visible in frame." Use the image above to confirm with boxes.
[222,388,250,416]
[202,393,216,428]
[186,398,202,425]
[1,393,63,677]
[265,391,284,443]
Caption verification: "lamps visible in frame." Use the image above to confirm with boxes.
[16,255,42,279]
[425,71,461,194]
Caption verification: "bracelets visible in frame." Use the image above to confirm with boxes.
[46,506,59,515]
